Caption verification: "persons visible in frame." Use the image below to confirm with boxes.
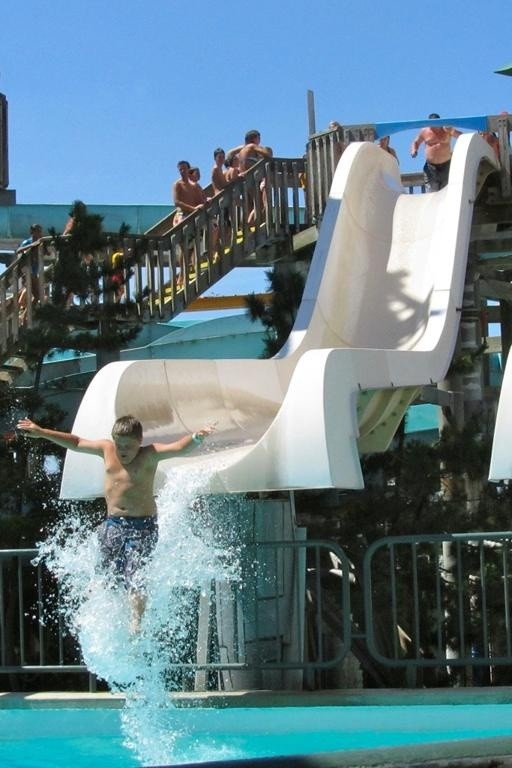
[171,131,274,284]
[298,113,500,227]
[15,415,217,635]
[16,200,134,322]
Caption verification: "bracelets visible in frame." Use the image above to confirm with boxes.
[192,433,206,446]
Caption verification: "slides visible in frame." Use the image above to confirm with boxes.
[58,131,502,501]
